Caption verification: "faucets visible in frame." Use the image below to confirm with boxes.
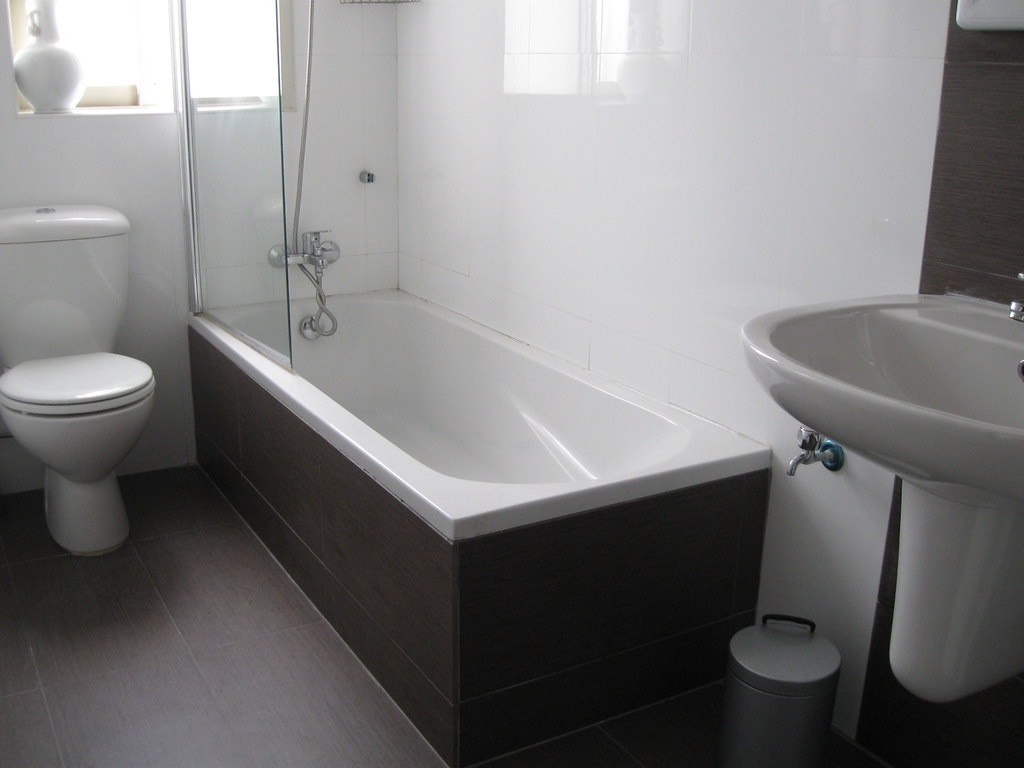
[785,427,845,476]
[267,229,340,270]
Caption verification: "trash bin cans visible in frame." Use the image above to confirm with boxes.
[717,613,842,768]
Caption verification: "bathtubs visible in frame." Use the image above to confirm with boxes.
[187,287,775,767]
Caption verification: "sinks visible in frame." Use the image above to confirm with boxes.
[741,293,1024,514]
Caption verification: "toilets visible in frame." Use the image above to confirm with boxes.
[2,206,156,558]
